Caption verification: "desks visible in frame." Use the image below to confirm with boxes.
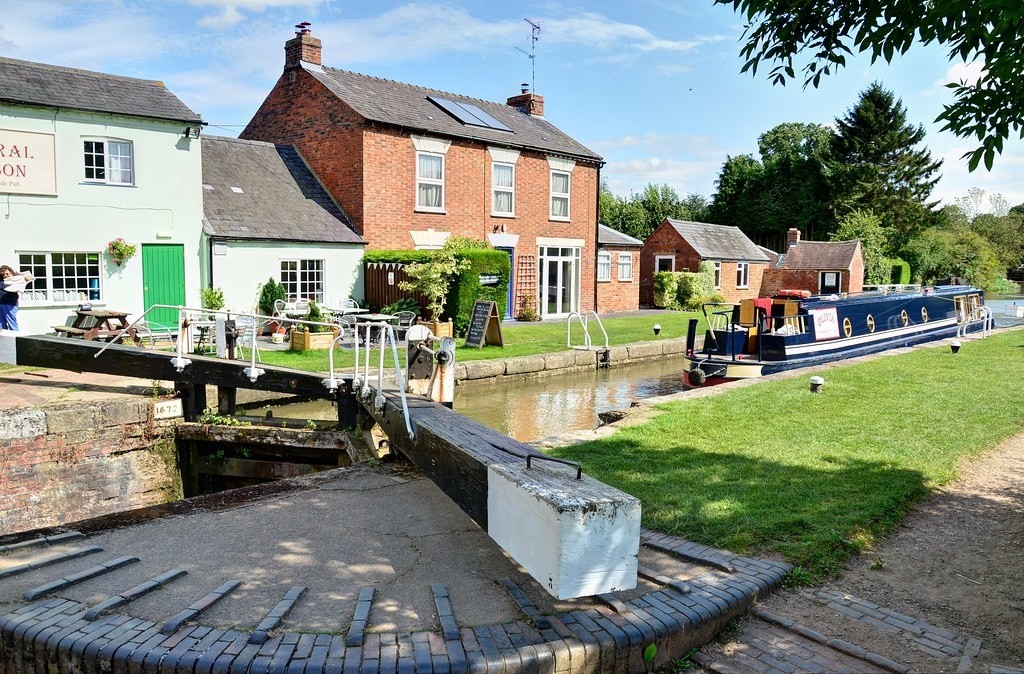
[281,308,329,332]
[356,313,399,346]
[66,308,132,344]
[330,307,369,341]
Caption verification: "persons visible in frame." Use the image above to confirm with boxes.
[0,264,34,331]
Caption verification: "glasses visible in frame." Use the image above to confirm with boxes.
[2,273,9,275]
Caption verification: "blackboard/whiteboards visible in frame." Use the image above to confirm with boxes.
[463,298,504,348]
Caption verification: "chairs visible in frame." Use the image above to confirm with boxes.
[235,311,261,363]
[293,298,311,332]
[260,299,291,334]
[135,321,174,352]
[189,315,213,353]
[338,299,359,341]
[388,311,416,343]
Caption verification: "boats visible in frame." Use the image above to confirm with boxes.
[683,285,995,388]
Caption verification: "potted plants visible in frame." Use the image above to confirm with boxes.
[257,326,264,336]
[397,253,471,339]
[290,300,339,351]
[259,276,286,334]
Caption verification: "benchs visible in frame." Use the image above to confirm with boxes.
[113,323,150,346]
[50,324,88,337]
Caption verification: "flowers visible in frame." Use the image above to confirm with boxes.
[106,237,136,259]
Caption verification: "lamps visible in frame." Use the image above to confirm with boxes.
[951,340,961,354]
[653,324,661,335]
[183,127,200,139]
[810,376,824,394]
